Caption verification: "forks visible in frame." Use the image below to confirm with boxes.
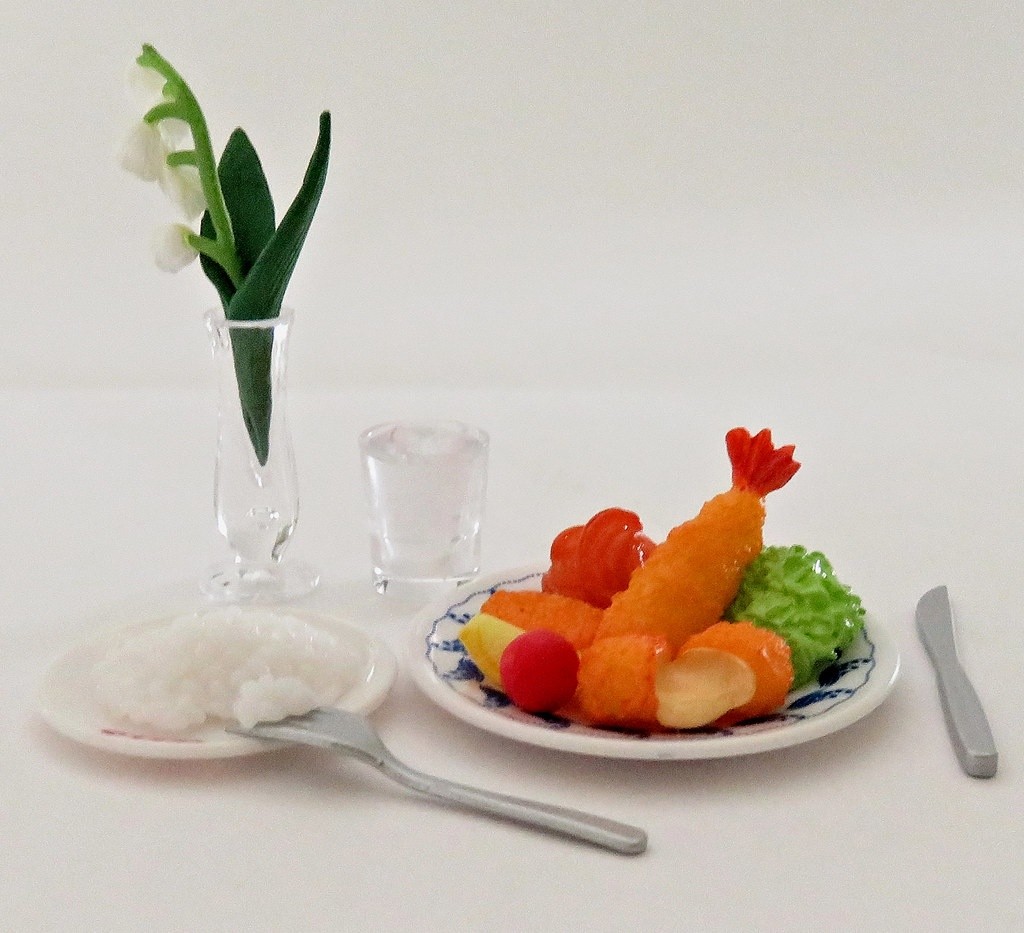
[224,707,648,855]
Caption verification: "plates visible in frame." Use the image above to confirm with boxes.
[405,563,902,757]
[39,604,400,760]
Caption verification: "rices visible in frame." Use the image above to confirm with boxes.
[77,605,353,731]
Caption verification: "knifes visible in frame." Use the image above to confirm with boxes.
[915,586,999,778]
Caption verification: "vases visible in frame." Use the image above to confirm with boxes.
[206,306,325,603]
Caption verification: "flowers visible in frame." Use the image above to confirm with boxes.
[116,39,333,467]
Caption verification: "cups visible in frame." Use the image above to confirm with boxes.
[361,418,494,594]
